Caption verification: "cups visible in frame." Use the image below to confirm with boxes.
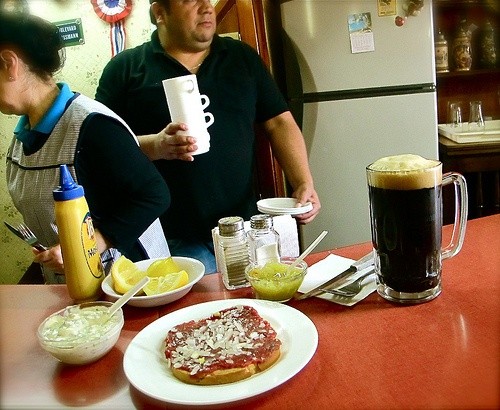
[365,157,468,303]
[447,101,463,133]
[467,101,486,131]
[162,73,214,156]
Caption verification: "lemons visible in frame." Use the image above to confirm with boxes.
[112,255,189,296]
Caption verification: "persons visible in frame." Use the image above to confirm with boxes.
[0,11,171,286]
[93,0,321,275]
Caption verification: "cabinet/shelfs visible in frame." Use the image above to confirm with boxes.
[432,0,500,226]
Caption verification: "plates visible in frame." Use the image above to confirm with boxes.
[122,299,319,405]
[256,197,313,215]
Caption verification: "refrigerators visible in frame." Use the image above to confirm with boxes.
[277,0,438,255]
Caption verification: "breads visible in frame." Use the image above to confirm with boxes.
[171,348,280,385]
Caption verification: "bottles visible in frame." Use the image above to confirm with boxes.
[216,216,251,290]
[52,164,105,303]
[246,215,281,264]
[478,20,498,67]
[451,29,473,71]
[434,32,449,73]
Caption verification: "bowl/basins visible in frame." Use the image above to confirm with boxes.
[36,301,124,365]
[101,256,205,307]
[244,256,308,302]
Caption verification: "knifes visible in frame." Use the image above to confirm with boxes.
[297,251,375,299]
[4,221,47,251]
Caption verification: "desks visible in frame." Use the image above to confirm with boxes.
[0,210,500,410]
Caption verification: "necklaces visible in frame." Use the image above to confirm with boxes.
[189,61,202,71]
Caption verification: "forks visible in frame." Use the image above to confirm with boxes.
[318,269,376,297]
[17,222,47,253]
